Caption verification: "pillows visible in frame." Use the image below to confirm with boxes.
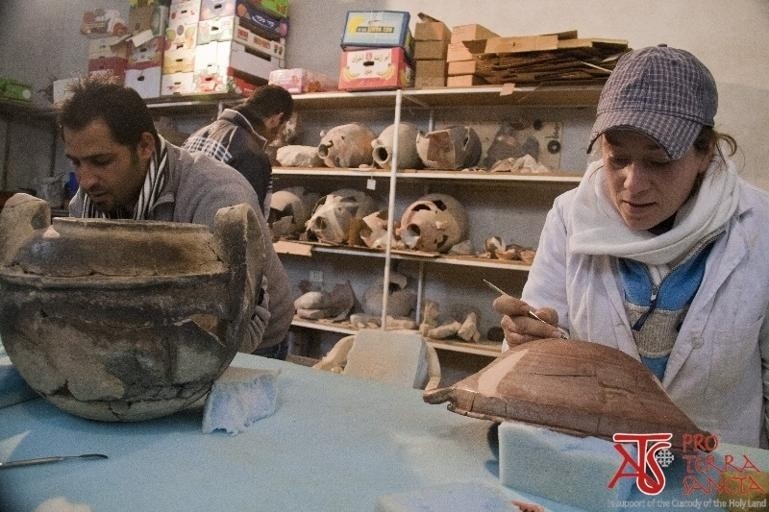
[341,329,429,391]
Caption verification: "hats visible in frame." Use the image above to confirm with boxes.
[585,43,719,162]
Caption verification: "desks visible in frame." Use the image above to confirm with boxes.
[0,347,767,511]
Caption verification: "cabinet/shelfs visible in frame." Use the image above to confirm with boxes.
[0,86,606,362]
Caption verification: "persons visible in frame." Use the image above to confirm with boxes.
[491,40,768,450]
[54,80,294,361]
[184,84,295,220]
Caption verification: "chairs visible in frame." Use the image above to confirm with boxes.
[307,329,442,394]
